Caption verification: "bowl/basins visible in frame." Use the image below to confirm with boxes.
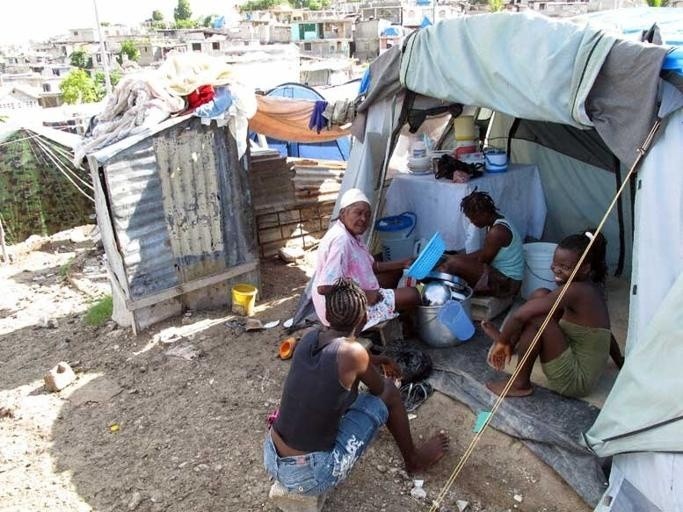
[421,282,453,307]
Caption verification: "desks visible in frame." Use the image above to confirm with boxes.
[393,162,535,248]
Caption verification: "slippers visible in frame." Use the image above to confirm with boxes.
[278,337,297,360]
[398,382,433,412]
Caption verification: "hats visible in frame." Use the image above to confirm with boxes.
[339,188,371,211]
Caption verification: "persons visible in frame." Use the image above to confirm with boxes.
[415,191,524,298]
[311,188,420,331]
[481,228,626,396]
[265,278,449,496]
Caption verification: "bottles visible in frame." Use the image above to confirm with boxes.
[414,141,426,159]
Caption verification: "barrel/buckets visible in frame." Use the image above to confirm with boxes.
[230,283,258,317]
[418,271,472,347]
[523,241,559,297]
[375,211,418,263]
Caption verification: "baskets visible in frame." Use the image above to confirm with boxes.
[404,231,446,281]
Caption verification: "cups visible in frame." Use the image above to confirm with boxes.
[441,301,475,341]
